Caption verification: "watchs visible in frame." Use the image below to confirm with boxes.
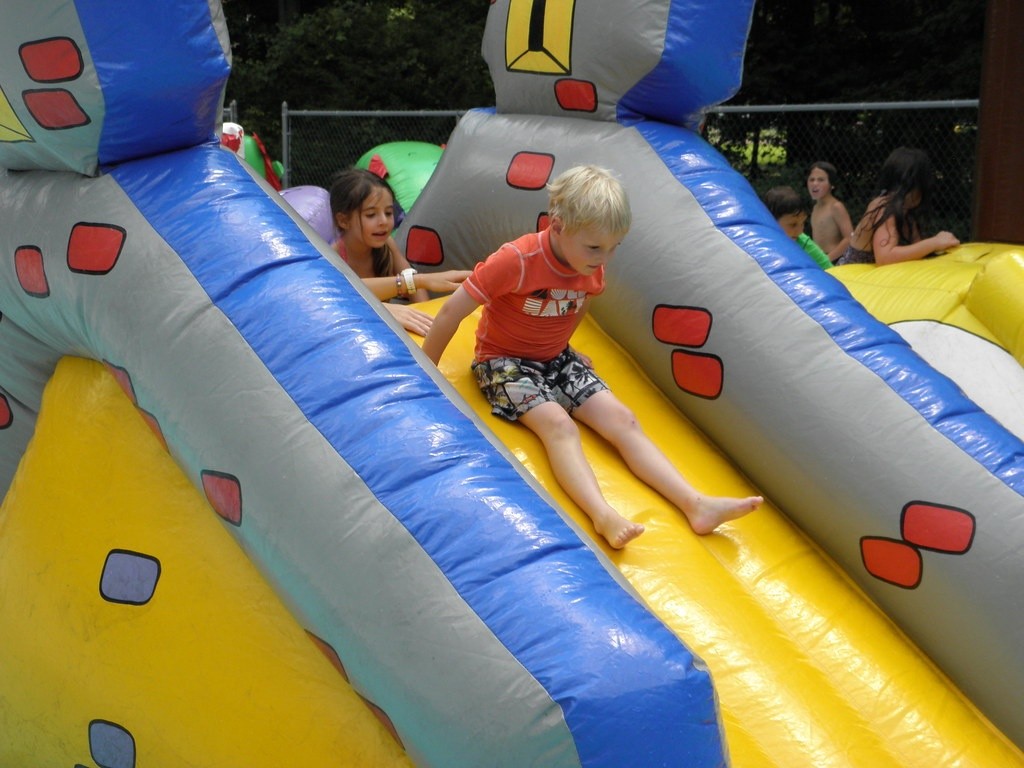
[401,268,418,295]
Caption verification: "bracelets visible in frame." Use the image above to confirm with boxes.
[397,273,404,299]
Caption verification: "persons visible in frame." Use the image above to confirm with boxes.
[328,169,474,339]
[763,186,834,271]
[421,164,764,551]
[807,162,853,261]
[835,144,960,267]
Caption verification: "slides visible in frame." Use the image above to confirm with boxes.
[379,284,1023,768]
